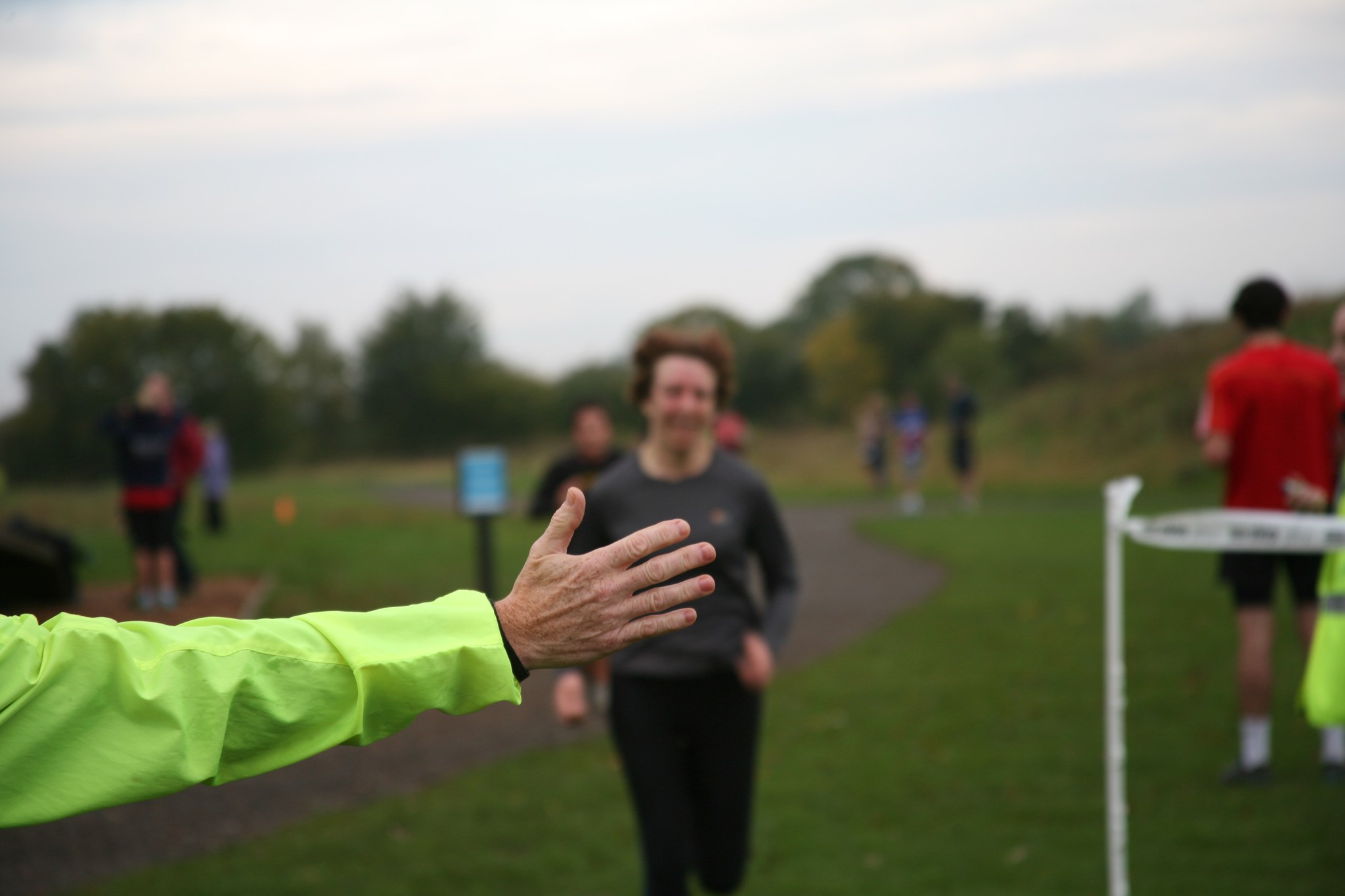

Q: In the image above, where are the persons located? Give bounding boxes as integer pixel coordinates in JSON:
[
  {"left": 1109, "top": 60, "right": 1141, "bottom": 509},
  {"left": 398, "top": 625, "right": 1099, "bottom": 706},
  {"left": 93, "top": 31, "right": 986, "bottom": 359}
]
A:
[
  {"left": 0, "top": 485, "right": 714, "bottom": 847},
  {"left": 856, "top": 386, "right": 893, "bottom": 503},
  {"left": 1201, "top": 278, "right": 1345, "bottom": 788},
  {"left": 197, "top": 416, "right": 230, "bottom": 537},
  {"left": 527, "top": 398, "right": 625, "bottom": 725},
  {"left": 896, "top": 387, "right": 928, "bottom": 519},
  {"left": 549, "top": 329, "right": 799, "bottom": 896},
  {"left": 943, "top": 371, "right": 982, "bottom": 507},
  {"left": 105, "top": 366, "right": 208, "bottom": 610}
]
